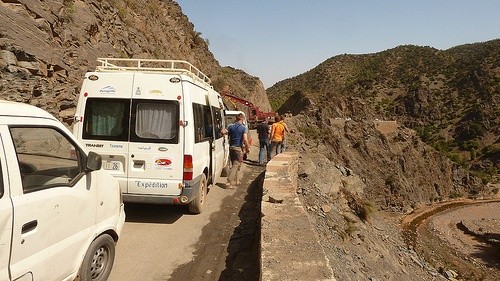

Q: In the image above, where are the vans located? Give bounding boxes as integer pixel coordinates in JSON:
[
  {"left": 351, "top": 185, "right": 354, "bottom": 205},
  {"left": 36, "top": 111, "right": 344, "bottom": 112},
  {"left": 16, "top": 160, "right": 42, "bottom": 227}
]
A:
[{"left": 73, "top": 57, "right": 230, "bottom": 213}]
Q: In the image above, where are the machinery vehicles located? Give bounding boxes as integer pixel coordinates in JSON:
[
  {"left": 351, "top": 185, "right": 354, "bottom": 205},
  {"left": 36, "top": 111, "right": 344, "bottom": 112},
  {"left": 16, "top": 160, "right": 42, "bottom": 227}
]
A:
[{"left": 218, "top": 89, "right": 279, "bottom": 129}]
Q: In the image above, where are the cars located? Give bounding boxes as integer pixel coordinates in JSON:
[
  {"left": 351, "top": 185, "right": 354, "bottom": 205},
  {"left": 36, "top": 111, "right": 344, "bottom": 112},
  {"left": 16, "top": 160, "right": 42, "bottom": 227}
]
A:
[
  {"left": 223, "top": 108, "right": 249, "bottom": 136},
  {"left": 0, "top": 97, "right": 126, "bottom": 281}
]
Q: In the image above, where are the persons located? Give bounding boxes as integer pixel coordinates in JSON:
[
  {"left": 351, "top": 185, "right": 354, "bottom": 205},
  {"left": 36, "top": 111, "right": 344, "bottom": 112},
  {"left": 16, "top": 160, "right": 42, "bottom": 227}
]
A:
[
  {"left": 221, "top": 114, "right": 251, "bottom": 189},
  {"left": 206, "top": 114, "right": 291, "bottom": 166}
]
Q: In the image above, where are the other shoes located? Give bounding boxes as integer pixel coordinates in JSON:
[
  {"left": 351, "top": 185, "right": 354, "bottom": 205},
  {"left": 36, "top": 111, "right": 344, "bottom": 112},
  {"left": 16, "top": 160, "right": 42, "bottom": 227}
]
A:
[{"left": 224, "top": 184, "right": 236, "bottom": 189}]
